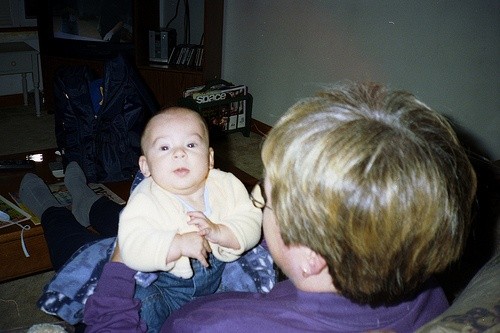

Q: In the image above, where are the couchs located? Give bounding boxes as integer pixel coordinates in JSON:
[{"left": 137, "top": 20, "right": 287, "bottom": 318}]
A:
[{"left": 411, "top": 252, "right": 500, "bottom": 333}]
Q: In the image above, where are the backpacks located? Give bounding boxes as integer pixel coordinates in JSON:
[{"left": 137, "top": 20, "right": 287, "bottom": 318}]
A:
[{"left": 48, "top": 59, "right": 158, "bottom": 182}]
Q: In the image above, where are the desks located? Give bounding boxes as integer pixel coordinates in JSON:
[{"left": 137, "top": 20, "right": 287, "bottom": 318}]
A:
[
  {"left": 0, "top": 43, "right": 41, "bottom": 118},
  {"left": 0, "top": 147, "right": 132, "bottom": 282}
]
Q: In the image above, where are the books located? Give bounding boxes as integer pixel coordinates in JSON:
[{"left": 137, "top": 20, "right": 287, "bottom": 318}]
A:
[{"left": 182, "top": 82, "right": 246, "bottom": 130}]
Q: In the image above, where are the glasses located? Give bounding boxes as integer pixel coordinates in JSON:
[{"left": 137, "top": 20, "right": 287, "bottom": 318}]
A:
[{"left": 249, "top": 179, "right": 275, "bottom": 212}]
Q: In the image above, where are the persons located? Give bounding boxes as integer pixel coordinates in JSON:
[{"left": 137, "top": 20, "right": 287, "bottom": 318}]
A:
[
  {"left": 118, "top": 108, "right": 263, "bottom": 333},
  {"left": 18, "top": 80, "right": 477, "bottom": 333}
]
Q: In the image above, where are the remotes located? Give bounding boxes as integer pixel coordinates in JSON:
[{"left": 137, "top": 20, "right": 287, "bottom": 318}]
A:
[{"left": 0, "top": 159, "right": 36, "bottom": 169}]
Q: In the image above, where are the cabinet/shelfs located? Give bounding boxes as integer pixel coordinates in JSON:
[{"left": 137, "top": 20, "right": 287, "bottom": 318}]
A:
[{"left": 132, "top": 1, "right": 224, "bottom": 108}]
